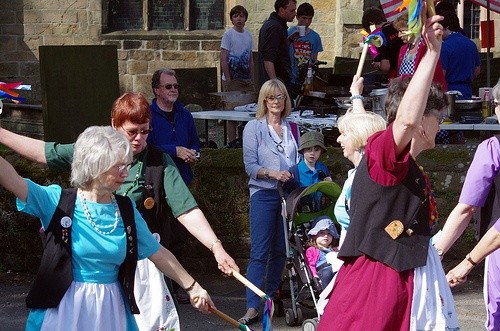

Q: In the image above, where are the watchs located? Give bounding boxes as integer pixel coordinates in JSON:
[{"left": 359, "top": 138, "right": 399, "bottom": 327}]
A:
[{"left": 434, "top": 244, "right": 444, "bottom": 256}]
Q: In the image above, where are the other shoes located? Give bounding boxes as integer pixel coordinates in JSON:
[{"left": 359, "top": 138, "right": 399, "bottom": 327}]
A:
[
  {"left": 175, "top": 286, "right": 191, "bottom": 304},
  {"left": 238, "top": 312, "right": 260, "bottom": 326}
]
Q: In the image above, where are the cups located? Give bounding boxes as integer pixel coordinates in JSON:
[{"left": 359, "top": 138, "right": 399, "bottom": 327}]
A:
[
  {"left": 443, "top": 91, "right": 458, "bottom": 116},
  {"left": 297, "top": 26, "right": 306, "bottom": 36},
  {"left": 370, "top": 89, "right": 389, "bottom": 120}
]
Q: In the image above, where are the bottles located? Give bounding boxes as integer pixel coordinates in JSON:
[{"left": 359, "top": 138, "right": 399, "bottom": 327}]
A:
[
  {"left": 482, "top": 89, "right": 494, "bottom": 121},
  {"left": 303, "top": 68, "right": 313, "bottom": 96}
]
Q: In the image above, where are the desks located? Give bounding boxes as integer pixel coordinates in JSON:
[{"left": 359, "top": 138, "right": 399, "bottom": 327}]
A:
[{"left": 188, "top": 108, "right": 500, "bottom": 147}]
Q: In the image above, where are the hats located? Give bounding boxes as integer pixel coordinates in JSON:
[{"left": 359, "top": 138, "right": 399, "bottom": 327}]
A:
[
  {"left": 297, "top": 132, "right": 327, "bottom": 156},
  {"left": 307, "top": 215, "right": 340, "bottom": 240}
]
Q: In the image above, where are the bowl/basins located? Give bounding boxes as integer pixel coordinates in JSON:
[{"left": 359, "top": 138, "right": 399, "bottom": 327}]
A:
[
  {"left": 454, "top": 100, "right": 483, "bottom": 110},
  {"left": 332, "top": 97, "right": 369, "bottom": 109},
  {"left": 309, "top": 92, "right": 326, "bottom": 98}
]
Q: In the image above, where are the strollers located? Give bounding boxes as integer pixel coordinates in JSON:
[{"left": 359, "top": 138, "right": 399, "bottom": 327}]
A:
[{"left": 277, "top": 170, "right": 342, "bottom": 331}]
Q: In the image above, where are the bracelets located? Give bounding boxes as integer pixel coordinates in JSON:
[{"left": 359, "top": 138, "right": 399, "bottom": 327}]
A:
[
  {"left": 265, "top": 169, "right": 269, "bottom": 179},
  {"left": 466, "top": 254, "right": 477, "bottom": 266},
  {"left": 185, "top": 280, "right": 196, "bottom": 292},
  {"left": 289, "top": 37, "right": 294, "bottom": 43},
  {"left": 351, "top": 95, "right": 364, "bottom": 103},
  {"left": 210, "top": 240, "right": 221, "bottom": 251}
]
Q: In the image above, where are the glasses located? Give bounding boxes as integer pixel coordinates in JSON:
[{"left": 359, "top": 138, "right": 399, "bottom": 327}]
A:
[
  {"left": 121, "top": 124, "right": 153, "bottom": 137},
  {"left": 161, "top": 83, "right": 182, "bottom": 89},
  {"left": 115, "top": 162, "right": 134, "bottom": 173},
  {"left": 265, "top": 94, "right": 287, "bottom": 103},
  {"left": 430, "top": 111, "right": 449, "bottom": 124},
  {"left": 491, "top": 99, "right": 500, "bottom": 110}
]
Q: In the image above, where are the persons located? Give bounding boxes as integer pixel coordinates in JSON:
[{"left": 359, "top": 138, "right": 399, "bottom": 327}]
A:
[
  {"left": 0, "top": 125, "right": 217, "bottom": 331},
  {"left": 316, "top": 3, "right": 450, "bottom": 331},
  {"left": 393, "top": 19, "right": 448, "bottom": 93},
  {"left": 283, "top": 131, "right": 332, "bottom": 213},
  {"left": 220, "top": 5, "right": 255, "bottom": 141},
  {"left": 148, "top": 69, "right": 202, "bottom": 303},
  {"left": 306, "top": 216, "right": 339, "bottom": 290},
  {"left": 433, "top": 77, "right": 500, "bottom": 331},
  {"left": 438, "top": 12, "right": 482, "bottom": 101},
  {"left": 334, "top": 76, "right": 460, "bottom": 330},
  {"left": 0, "top": 92, "right": 240, "bottom": 275},
  {"left": 287, "top": 2, "right": 323, "bottom": 84},
  {"left": 445, "top": 217, "right": 500, "bottom": 287},
  {"left": 362, "top": 7, "right": 406, "bottom": 87},
  {"left": 237, "top": 80, "right": 300, "bottom": 324},
  {"left": 259, "top": 0, "right": 300, "bottom": 92}
]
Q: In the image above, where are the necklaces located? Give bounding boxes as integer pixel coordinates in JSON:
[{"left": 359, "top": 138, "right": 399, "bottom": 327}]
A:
[
  {"left": 266, "top": 115, "right": 284, "bottom": 153},
  {"left": 76, "top": 189, "right": 119, "bottom": 235},
  {"left": 123, "top": 162, "right": 142, "bottom": 196}
]
